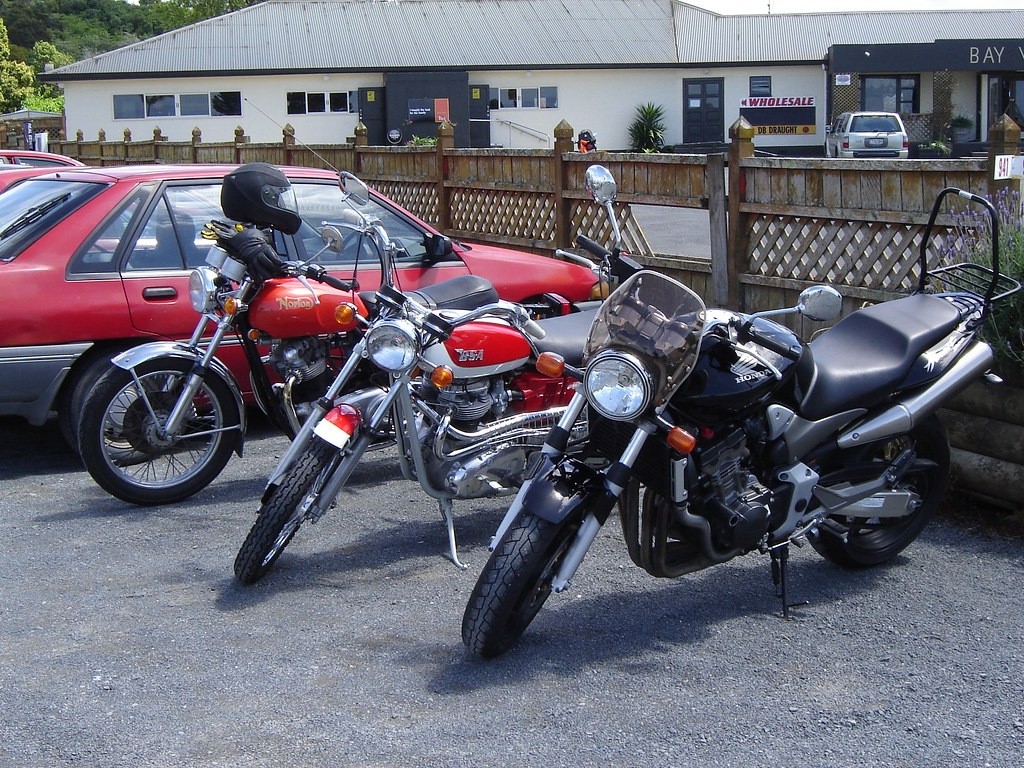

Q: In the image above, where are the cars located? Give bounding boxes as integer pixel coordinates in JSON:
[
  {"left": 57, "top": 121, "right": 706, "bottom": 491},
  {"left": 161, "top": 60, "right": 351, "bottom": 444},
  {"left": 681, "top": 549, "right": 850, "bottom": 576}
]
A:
[
  {"left": 0, "top": 157, "right": 611, "bottom": 472},
  {"left": 0, "top": 148, "right": 238, "bottom": 256},
  {"left": 823, "top": 110, "right": 915, "bottom": 161}
]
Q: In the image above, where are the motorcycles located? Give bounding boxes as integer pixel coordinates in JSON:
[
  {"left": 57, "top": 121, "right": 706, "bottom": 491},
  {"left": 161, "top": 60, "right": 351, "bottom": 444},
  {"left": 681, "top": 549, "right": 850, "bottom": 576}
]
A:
[
  {"left": 232, "top": 169, "right": 671, "bottom": 590},
  {"left": 459, "top": 172, "right": 1022, "bottom": 658},
  {"left": 75, "top": 160, "right": 500, "bottom": 507}
]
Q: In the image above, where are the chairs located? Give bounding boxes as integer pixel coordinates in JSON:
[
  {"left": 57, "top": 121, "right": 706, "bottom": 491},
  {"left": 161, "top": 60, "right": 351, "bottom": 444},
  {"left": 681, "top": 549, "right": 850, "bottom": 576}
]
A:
[{"left": 149, "top": 211, "right": 204, "bottom": 266}]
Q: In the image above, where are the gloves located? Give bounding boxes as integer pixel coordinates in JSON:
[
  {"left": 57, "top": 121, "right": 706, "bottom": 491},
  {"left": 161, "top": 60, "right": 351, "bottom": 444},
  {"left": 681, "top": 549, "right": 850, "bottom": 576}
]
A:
[
  {"left": 201, "top": 220, "right": 244, "bottom": 240},
  {"left": 227, "top": 229, "right": 283, "bottom": 282}
]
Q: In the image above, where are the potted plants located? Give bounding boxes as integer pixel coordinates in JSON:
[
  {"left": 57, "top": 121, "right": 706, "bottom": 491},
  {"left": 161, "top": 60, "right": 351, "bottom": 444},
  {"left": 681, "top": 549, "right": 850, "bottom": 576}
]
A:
[{"left": 916, "top": 115, "right": 976, "bottom": 158}]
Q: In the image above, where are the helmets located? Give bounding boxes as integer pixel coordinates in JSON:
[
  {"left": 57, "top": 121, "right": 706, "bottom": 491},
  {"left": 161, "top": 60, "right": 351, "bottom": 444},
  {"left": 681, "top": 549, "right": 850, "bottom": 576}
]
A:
[{"left": 220, "top": 162, "right": 303, "bottom": 235}]
